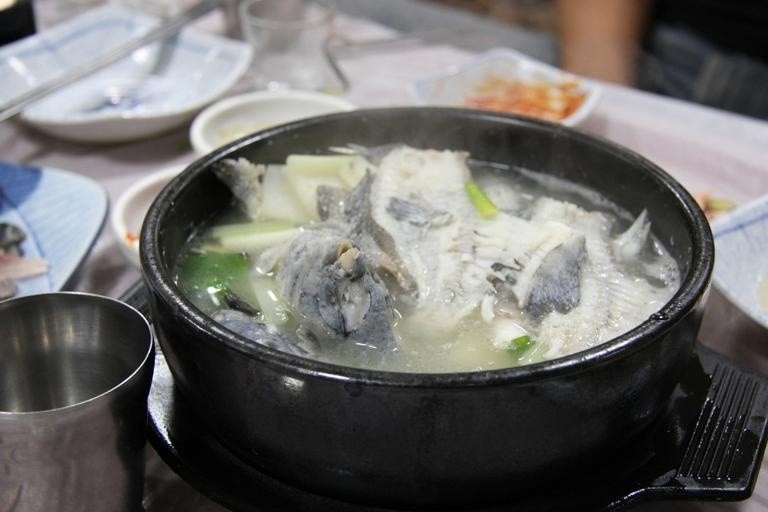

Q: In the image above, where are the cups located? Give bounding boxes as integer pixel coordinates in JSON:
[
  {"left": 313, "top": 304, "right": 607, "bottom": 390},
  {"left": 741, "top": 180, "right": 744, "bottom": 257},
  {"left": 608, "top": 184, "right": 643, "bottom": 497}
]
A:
[{"left": 0, "top": 293, "right": 156, "bottom": 512}]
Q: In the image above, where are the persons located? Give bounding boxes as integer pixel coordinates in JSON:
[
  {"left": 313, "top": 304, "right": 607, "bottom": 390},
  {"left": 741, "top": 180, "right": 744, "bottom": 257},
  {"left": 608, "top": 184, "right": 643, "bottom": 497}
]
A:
[{"left": 560, "top": 0, "right": 768, "bottom": 119}]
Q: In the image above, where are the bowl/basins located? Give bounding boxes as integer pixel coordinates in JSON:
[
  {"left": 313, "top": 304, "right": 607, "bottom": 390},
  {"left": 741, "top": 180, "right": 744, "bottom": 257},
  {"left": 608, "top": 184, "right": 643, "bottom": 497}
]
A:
[{"left": 140, "top": 104, "right": 718, "bottom": 504}]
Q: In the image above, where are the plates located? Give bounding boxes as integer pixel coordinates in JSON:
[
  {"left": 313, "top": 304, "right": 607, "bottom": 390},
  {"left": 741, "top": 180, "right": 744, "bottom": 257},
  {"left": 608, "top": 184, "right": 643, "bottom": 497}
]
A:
[
  {"left": 188, "top": 94, "right": 363, "bottom": 155},
  {"left": 416, "top": 49, "right": 606, "bottom": 128},
  {"left": 2, "top": 5, "right": 254, "bottom": 152},
  {"left": 100, "top": 277, "right": 768, "bottom": 512},
  {"left": 705, "top": 193, "right": 766, "bottom": 328},
  {"left": 1, "top": 163, "right": 108, "bottom": 298},
  {"left": 109, "top": 164, "right": 181, "bottom": 264}
]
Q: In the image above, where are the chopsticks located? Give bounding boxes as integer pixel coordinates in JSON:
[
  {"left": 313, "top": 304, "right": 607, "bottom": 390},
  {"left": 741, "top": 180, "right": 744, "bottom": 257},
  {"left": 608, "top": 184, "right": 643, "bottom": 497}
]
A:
[{"left": 1, "top": 5, "right": 243, "bottom": 128}]
